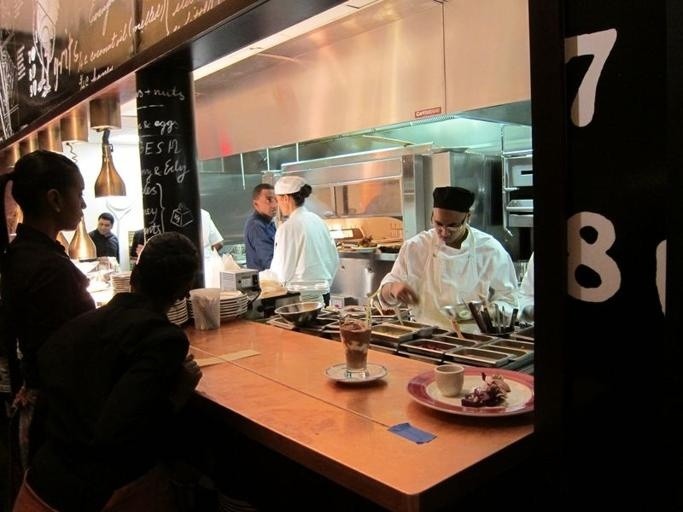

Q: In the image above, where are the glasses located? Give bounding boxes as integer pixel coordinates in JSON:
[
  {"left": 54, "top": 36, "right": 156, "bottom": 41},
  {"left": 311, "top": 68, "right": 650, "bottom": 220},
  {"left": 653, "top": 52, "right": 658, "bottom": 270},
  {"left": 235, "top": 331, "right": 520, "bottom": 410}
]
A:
[{"left": 429, "top": 210, "right": 468, "bottom": 231}]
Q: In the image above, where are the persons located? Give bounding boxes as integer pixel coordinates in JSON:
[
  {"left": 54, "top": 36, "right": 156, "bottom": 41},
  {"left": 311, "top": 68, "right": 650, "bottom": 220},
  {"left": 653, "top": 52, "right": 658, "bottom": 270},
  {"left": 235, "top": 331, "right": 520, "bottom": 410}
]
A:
[
  {"left": 88, "top": 212, "right": 120, "bottom": 265},
  {"left": 519, "top": 250, "right": 535, "bottom": 321},
  {"left": 131, "top": 228, "right": 145, "bottom": 257},
  {"left": 1, "top": 148, "right": 95, "bottom": 473},
  {"left": 243, "top": 183, "right": 280, "bottom": 272},
  {"left": 200, "top": 208, "right": 226, "bottom": 289},
  {"left": 376, "top": 186, "right": 519, "bottom": 329},
  {"left": 258, "top": 175, "right": 340, "bottom": 308},
  {"left": 7, "top": 231, "right": 203, "bottom": 512}
]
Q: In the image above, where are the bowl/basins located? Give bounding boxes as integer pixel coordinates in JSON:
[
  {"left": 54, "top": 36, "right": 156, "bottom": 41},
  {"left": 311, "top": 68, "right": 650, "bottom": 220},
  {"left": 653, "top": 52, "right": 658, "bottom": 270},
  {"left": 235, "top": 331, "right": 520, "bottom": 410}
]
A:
[{"left": 273, "top": 301, "right": 326, "bottom": 325}]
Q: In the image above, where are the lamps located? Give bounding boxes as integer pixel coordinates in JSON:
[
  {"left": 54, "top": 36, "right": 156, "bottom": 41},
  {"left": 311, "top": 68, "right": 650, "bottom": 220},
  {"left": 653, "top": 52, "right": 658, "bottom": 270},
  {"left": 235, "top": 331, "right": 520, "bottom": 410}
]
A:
[
  {"left": 68, "top": 214, "right": 97, "bottom": 261},
  {"left": 56, "top": 232, "right": 69, "bottom": 256},
  {"left": 94, "top": 128, "right": 127, "bottom": 197}
]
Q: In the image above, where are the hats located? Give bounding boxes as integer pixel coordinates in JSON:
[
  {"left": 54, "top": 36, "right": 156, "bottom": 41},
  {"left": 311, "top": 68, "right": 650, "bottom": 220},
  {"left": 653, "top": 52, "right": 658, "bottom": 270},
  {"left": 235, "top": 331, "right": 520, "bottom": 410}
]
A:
[
  {"left": 272, "top": 174, "right": 307, "bottom": 196},
  {"left": 432, "top": 185, "right": 475, "bottom": 213}
]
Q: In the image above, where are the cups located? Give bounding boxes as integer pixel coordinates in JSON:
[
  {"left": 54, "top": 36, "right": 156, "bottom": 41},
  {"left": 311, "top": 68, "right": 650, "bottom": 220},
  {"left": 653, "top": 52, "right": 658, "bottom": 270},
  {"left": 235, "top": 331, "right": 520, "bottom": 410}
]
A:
[
  {"left": 433, "top": 363, "right": 466, "bottom": 398},
  {"left": 188, "top": 286, "right": 221, "bottom": 331},
  {"left": 337, "top": 304, "right": 373, "bottom": 378}
]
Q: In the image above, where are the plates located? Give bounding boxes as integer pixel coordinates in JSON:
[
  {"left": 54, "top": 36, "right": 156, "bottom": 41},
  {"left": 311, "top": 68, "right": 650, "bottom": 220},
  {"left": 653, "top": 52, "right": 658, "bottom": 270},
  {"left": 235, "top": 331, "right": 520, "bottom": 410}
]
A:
[
  {"left": 351, "top": 244, "right": 381, "bottom": 253},
  {"left": 109, "top": 270, "right": 248, "bottom": 326},
  {"left": 403, "top": 365, "right": 537, "bottom": 423},
  {"left": 324, "top": 363, "right": 387, "bottom": 385}
]
